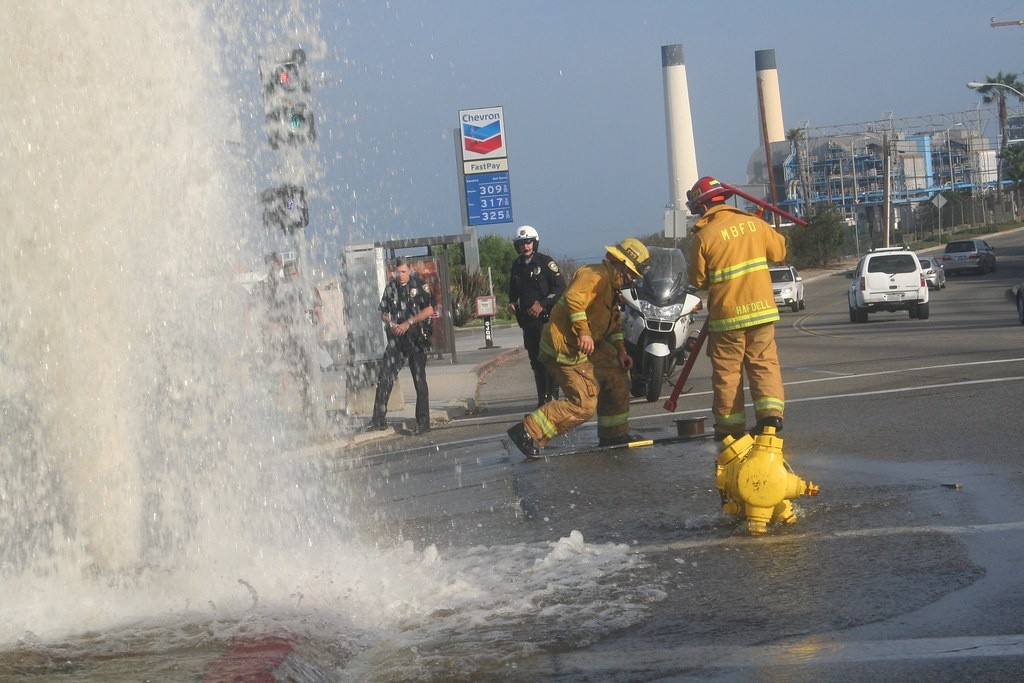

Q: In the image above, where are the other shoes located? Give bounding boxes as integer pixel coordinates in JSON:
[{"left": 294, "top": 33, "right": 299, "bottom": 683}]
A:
[
  {"left": 749, "top": 416, "right": 783, "bottom": 436},
  {"left": 363, "top": 418, "right": 388, "bottom": 431},
  {"left": 714, "top": 432, "right": 745, "bottom": 440},
  {"left": 538, "top": 402, "right": 545, "bottom": 407},
  {"left": 412, "top": 423, "right": 430, "bottom": 436}
]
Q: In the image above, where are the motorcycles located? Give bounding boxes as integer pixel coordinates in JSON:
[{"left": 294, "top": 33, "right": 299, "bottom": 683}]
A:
[{"left": 617, "top": 245, "right": 703, "bottom": 402}]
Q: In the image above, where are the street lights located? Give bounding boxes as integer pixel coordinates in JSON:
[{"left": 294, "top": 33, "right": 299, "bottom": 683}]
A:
[{"left": 946, "top": 123, "right": 963, "bottom": 192}]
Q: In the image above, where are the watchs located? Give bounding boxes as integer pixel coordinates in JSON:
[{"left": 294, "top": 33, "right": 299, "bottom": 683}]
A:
[{"left": 406, "top": 318, "right": 414, "bottom": 326}]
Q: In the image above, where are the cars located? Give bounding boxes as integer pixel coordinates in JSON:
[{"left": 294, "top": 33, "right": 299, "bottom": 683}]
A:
[{"left": 915, "top": 255, "right": 946, "bottom": 291}]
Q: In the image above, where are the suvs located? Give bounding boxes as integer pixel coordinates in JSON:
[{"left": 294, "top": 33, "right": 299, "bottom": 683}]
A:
[
  {"left": 768, "top": 263, "right": 806, "bottom": 312},
  {"left": 846, "top": 246, "right": 930, "bottom": 323},
  {"left": 943, "top": 238, "right": 997, "bottom": 278}
]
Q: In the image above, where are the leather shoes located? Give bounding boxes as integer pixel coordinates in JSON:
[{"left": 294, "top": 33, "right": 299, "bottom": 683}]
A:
[
  {"left": 507, "top": 423, "right": 539, "bottom": 455},
  {"left": 598, "top": 434, "right": 644, "bottom": 447}
]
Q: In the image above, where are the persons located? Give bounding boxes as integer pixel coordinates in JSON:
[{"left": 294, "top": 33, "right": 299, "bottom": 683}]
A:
[
  {"left": 685, "top": 176, "right": 787, "bottom": 441},
  {"left": 507, "top": 225, "right": 568, "bottom": 418},
  {"left": 356, "top": 255, "right": 436, "bottom": 436},
  {"left": 250, "top": 251, "right": 338, "bottom": 394},
  {"left": 507, "top": 237, "right": 654, "bottom": 458}
]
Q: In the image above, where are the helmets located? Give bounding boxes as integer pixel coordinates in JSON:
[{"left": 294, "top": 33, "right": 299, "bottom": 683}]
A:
[
  {"left": 513, "top": 226, "right": 539, "bottom": 254},
  {"left": 605, "top": 238, "right": 651, "bottom": 278},
  {"left": 685, "top": 176, "right": 734, "bottom": 215}
]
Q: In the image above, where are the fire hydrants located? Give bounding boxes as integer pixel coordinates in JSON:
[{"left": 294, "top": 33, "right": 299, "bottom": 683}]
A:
[{"left": 714, "top": 425, "right": 820, "bottom": 537}]
